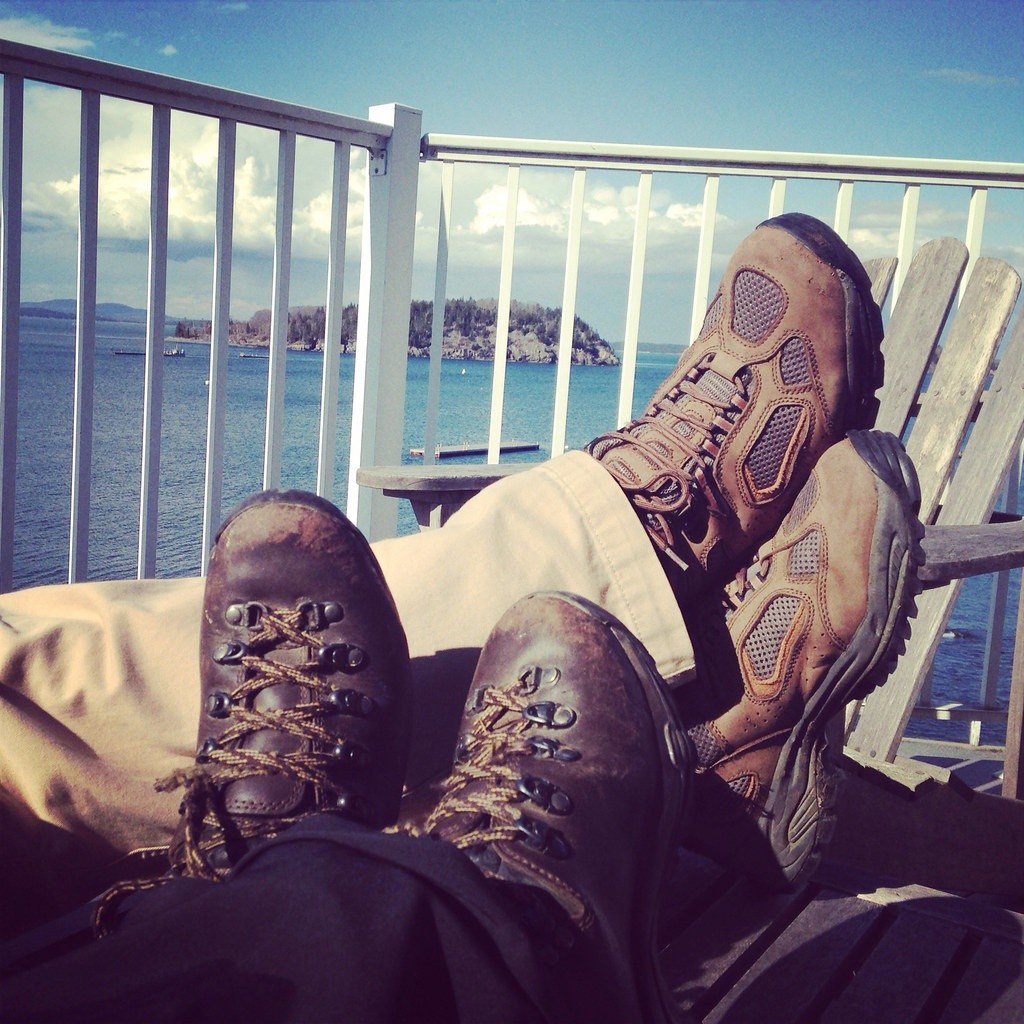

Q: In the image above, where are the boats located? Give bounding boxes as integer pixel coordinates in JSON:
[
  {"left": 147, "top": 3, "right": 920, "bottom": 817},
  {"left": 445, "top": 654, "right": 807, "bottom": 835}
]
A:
[
  {"left": 941, "top": 629, "right": 970, "bottom": 638},
  {"left": 237, "top": 353, "right": 270, "bottom": 359},
  {"left": 113, "top": 346, "right": 187, "bottom": 358}
]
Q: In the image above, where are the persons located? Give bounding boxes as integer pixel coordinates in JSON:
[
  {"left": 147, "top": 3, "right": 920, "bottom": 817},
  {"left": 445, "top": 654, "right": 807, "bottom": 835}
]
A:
[
  {"left": 1, "top": 490, "right": 702, "bottom": 1024},
  {"left": 1, "top": 212, "right": 926, "bottom": 896}
]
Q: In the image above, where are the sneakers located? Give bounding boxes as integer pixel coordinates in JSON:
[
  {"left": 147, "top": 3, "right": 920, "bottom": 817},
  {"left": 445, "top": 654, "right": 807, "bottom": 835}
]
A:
[
  {"left": 583, "top": 212, "right": 885, "bottom": 619},
  {"left": 664, "top": 428, "right": 926, "bottom": 897}
]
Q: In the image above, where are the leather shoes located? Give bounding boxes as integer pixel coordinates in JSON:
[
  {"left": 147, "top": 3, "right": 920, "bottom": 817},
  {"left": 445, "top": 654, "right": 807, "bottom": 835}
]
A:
[
  {"left": 90, "top": 496, "right": 414, "bottom": 941},
  {"left": 382, "top": 589, "right": 693, "bottom": 1024}
]
